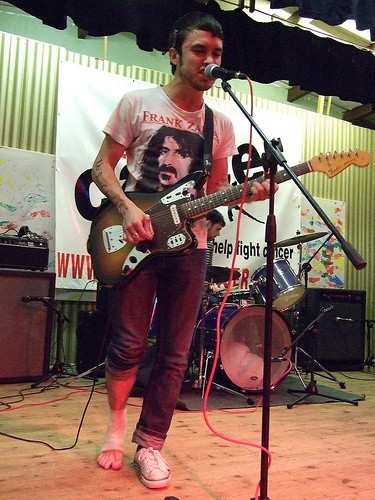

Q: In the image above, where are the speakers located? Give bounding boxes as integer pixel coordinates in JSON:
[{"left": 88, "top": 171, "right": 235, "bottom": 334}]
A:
[
  {"left": 75, "top": 309, "right": 105, "bottom": 380},
  {"left": 0, "top": 267, "right": 56, "bottom": 383},
  {"left": 285, "top": 287, "right": 366, "bottom": 373}
]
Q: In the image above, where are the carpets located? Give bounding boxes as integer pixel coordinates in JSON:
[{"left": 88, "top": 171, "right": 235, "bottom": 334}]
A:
[{"left": 131, "top": 374, "right": 365, "bottom": 411}]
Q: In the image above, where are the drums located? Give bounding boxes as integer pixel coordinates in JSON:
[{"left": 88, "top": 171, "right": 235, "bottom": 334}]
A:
[
  {"left": 250, "top": 257, "right": 306, "bottom": 313},
  {"left": 192, "top": 301, "right": 294, "bottom": 392},
  {"left": 217, "top": 287, "right": 254, "bottom": 302}
]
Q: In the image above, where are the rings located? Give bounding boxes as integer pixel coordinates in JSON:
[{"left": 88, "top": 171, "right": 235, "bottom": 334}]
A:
[{"left": 133, "top": 233, "right": 138, "bottom": 239}]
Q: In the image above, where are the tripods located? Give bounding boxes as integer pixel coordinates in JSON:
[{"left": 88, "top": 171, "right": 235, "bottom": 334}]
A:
[
  {"left": 31, "top": 301, "right": 99, "bottom": 389},
  {"left": 182, "top": 290, "right": 256, "bottom": 406},
  {"left": 279, "top": 243, "right": 359, "bottom": 410}
]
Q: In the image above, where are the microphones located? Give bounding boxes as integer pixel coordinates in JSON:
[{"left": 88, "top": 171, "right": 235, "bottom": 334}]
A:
[
  {"left": 270, "top": 357, "right": 287, "bottom": 362},
  {"left": 21, "top": 295, "right": 53, "bottom": 303},
  {"left": 204, "top": 64, "right": 247, "bottom": 79},
  {"left": 335, "top": 316, "right": 355, "bottom": 322}
]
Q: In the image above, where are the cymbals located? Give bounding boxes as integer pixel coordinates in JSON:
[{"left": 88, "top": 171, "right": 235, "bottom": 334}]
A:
[
  {"left": 274, "top": 229, "right": 328, "bottom": 249},
  {"left": 204, "top": 262, "right": 243, "bottom": 284}
]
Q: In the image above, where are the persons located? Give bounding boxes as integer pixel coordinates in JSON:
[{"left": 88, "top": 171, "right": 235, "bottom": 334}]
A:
[
  {"left": 91, "top": 11, "right": 278, "bottom": 489},
  {"left": 206, "top": 210, "right": 225, "bottom": 280}
]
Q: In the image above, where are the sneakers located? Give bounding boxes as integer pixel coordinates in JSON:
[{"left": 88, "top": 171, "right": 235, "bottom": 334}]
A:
[{"left": 133, "top": 447, "right": 172, "bottom": 488}]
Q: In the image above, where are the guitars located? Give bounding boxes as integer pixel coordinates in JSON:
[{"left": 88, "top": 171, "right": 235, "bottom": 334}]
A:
[{"left": 88, "top": 146, "right": 372, "bottom": 288}]
